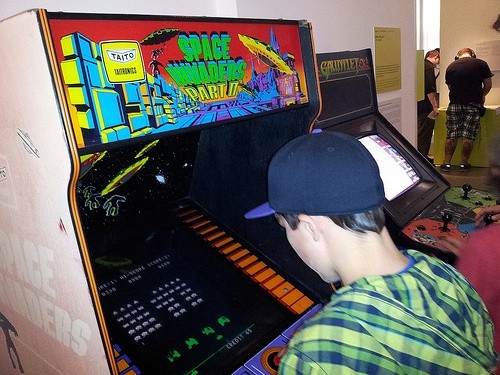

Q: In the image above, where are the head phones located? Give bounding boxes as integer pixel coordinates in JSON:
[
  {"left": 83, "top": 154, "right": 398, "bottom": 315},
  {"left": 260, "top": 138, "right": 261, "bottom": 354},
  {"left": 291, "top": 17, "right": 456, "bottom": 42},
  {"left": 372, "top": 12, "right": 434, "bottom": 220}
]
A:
[{"left": 455, "top": 51, "right": 476, "bottom": 60}]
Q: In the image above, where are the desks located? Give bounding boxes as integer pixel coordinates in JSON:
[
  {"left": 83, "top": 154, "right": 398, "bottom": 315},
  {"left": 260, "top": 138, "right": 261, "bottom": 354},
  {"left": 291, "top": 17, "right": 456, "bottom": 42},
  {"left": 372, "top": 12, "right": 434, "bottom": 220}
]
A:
[{"left": 434, "top": 106, "right": 500, "bottom": 167}]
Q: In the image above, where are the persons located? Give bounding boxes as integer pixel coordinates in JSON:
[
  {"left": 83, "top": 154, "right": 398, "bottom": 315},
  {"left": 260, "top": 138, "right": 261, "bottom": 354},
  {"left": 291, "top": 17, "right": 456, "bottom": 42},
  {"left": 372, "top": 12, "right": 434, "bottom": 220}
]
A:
[
  {"left": 417, "top": 50, "right": 439, "bottom": 164},
  {"left": 245, "top": 129, "right": 500, "bottom": 375},
  {"left": 440, "top": 48, "right": 495, "bottom": 173},
  {"left": 433, "top": 48, "right": 440, "bottom": 106},
  {"left": 436, "top": 136, "right": 500, "bottom": 375}
]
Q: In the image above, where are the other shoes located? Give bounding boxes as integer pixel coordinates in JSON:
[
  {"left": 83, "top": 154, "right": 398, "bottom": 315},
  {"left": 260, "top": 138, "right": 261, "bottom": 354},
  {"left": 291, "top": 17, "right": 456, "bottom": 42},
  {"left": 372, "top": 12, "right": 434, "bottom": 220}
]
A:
[
  {"left": 459, "top": 163, "right": 471, "bottom": 171},
  {"left": 440, "top": 163, "right": 451, "bottom": 172}
]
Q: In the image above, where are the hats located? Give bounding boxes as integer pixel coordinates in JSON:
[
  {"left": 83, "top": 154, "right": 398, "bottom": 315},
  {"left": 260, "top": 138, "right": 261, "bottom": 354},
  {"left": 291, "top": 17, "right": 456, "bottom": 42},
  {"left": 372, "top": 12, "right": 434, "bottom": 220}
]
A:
[{"left": 244, "top": 128, "right": 386, "bottom": 219}]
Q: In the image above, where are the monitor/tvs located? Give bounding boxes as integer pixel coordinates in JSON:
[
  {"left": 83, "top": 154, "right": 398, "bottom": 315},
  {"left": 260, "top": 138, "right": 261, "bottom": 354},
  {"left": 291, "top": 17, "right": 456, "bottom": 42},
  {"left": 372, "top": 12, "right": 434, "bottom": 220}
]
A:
[
  {"left": 357, "top": 134, "right": 420, "bottom": 201},
  {"left": 83, "top": 196, "right": 318, "bottom": 375}
]
